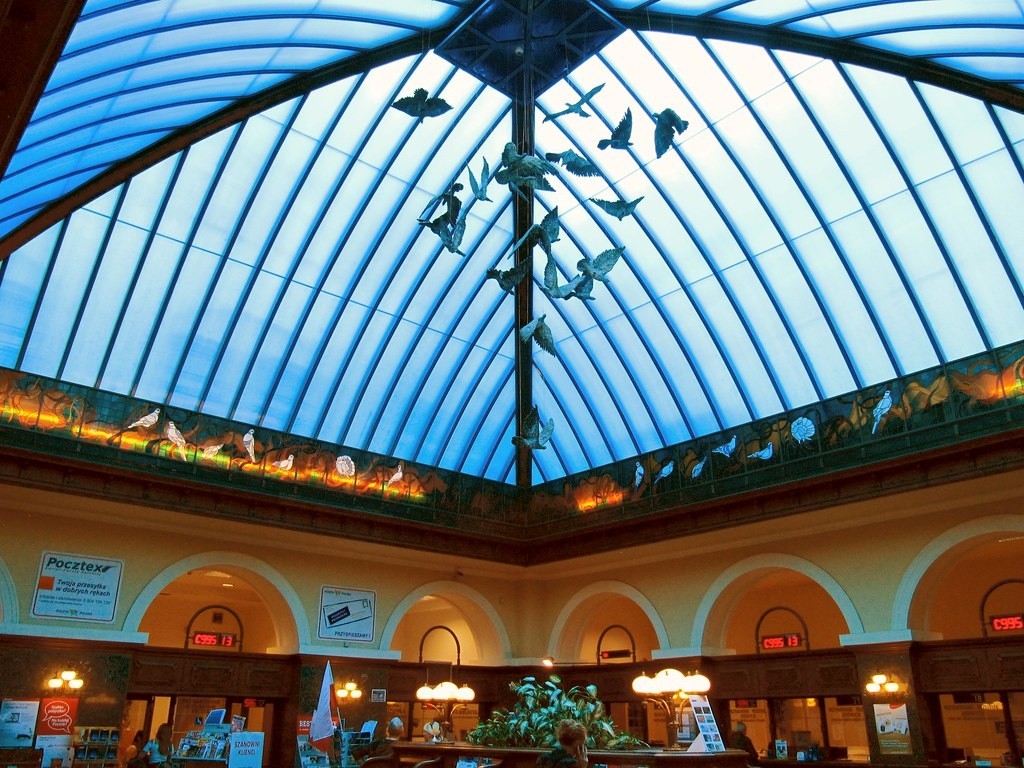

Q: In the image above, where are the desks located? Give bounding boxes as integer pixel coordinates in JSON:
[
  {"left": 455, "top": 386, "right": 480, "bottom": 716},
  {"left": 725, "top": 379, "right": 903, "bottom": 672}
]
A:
[{"left": 170, "top": 756, "right": 229, "bottom": 768}]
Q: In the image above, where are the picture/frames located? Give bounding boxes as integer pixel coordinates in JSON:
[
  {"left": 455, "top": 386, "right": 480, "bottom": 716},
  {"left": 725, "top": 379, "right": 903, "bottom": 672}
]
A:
[{"left": 371, "top": 688, "right": 387, "bottom": 702}]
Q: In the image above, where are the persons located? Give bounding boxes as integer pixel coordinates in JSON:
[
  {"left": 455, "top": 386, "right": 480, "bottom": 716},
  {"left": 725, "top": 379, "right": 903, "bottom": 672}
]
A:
[
  {"left": 534, "top": 719, "right": 587, "bottom": 768},
  {"left": 726, "top": 722, "right": 758, "bottom": 759},
  {"left": 351, "top": 717, "right": 403, "bottom": 766},
  {"left": 423, "top": 722, "right": 443, "bottom": 742},
  {"left": 122, "top": 723, "right": 174, "bottom": 768}
]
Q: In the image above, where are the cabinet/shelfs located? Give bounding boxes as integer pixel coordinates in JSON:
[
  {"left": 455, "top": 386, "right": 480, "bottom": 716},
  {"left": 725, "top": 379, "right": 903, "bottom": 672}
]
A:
[{"left": 73, "top": 727, "right": 118, "bottom": 768}]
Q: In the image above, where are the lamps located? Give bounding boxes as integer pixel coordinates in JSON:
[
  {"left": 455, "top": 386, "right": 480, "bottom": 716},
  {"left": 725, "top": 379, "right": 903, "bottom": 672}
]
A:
[
  {"left": 866, "top": 671, "right": 898, "bottom": 693},
  {"left": 416, "top": 681, "right": 474, "bottom": 744},
  {"left": 543, "top": 657, "right": 555, "bottom": 666},
  {"left": 48, "top": 664, "right": 83, "bottom": 689},
  {"left": 632, "top": 668, "right": 711, "bottom": 751},
  {"left": 337, "top": 678, "right": 362, "bottom": 698}
]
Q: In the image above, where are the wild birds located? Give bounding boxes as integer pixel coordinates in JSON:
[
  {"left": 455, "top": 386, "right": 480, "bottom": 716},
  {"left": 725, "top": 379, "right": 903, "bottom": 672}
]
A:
[
  {"left": 589, "top": 195, "right": 644, "bottom": 221},
  {"left": 597, "top": 108, "right": 633, "bottom": 150},
  {"left": 652, "top": 108, "right": 689, "bottom": 159},
  {"left": 415, "top": 142, "right": 626, "bottom": 300},
  {"left": 519, "top": 315, "right": 557, "bottom": 359},
  {"left": 390, "top": 88, "right": 452, "bottom": 123},
  {"left": 542, "top": 83, "right": 605, "bottom": 123},
  {"left": 511, "top": 405, "right": 555, "bottom": 449}
]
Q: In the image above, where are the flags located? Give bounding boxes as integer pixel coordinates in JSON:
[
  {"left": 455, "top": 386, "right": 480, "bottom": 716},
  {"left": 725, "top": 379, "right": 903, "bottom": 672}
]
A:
[{"left": 309, "top": 660, "right": 339, "bottom": 761}]
